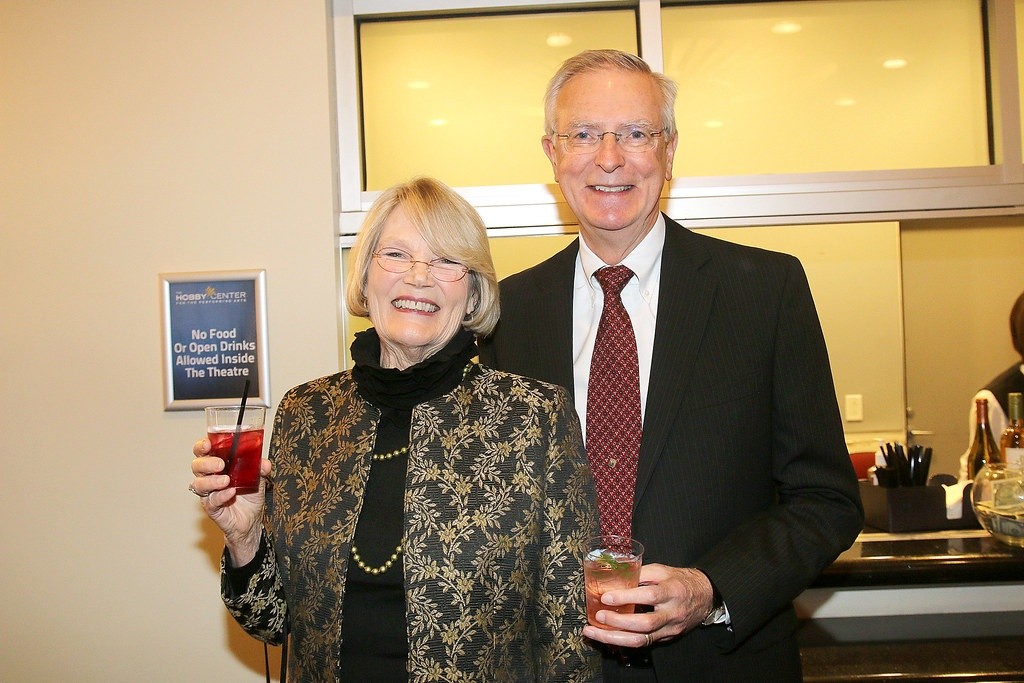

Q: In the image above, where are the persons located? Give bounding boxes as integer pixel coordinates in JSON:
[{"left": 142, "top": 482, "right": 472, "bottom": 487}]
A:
[
  {"left": 189, "top": 174, "right": 609, "bottom": 683},
  {"left": 957, "top": 294, "right": 1024, "bottom": 480},
  {"left": 477, "top": 48, "right": 865, "bottom": 683}
]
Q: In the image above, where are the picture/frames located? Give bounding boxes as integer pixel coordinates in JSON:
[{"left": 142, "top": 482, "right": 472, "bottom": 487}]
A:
[{"left": 159, "top": 269, "right": 271, "bottom": 412}]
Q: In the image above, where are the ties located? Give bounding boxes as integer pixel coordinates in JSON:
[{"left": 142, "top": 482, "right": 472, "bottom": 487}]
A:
[{"left": 586, "top": 265, "right": 643, "bottom": 550}]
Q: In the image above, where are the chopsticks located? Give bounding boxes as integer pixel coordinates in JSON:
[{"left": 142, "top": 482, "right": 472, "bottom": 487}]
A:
[{"left": 876, "top": 441, "right": 934, "bottom": 486}]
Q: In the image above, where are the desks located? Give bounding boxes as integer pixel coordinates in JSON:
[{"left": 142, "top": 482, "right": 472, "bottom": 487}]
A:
[{"left": 828, "top": 531, "right": 1024, "bottom": 587}]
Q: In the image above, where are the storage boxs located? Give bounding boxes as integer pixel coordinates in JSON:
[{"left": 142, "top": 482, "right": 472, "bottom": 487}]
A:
[{"left": 859, "top": 480, "right": 948, "bottom": 534}]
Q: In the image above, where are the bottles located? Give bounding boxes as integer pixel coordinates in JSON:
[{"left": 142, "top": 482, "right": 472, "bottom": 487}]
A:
[
  {"left": 1000, "top": 391, "right": 1024, "bottom": 465},
  {"left": 968, "top": 400, "right": 1001, "bottom": 480}
]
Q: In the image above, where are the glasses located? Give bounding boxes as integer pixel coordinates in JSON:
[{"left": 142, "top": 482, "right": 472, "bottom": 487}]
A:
[
  {"left": 373, "top": 247, "right": 474, "bottom": 282},
  {"left": 556, "top": 126, "right": 665, "bottom": 152}
]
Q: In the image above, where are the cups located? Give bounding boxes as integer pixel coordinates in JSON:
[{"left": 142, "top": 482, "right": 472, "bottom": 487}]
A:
[
  {"left": 583, "top": 535, "right": 645, "bottom": 631},
  {"left": 205, "top": 405, "right": 266, "bottom": 494}
]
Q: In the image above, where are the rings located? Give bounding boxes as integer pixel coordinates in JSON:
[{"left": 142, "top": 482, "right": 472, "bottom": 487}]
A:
[
  {"left": 189, "top": 488, "right": 209, "bottom": 497},
  {"left": 645, "top": 634, "right": 653, "bottom": 646}
]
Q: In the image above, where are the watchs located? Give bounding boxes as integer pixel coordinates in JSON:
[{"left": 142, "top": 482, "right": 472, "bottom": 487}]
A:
[{"left": 698, "top": 568, "right": 725, "bottom": 626}]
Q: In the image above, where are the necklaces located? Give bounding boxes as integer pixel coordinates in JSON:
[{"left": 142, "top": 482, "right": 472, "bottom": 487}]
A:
[{"left": 347, "top": 352, "right": 477, "bottom": 577}]
